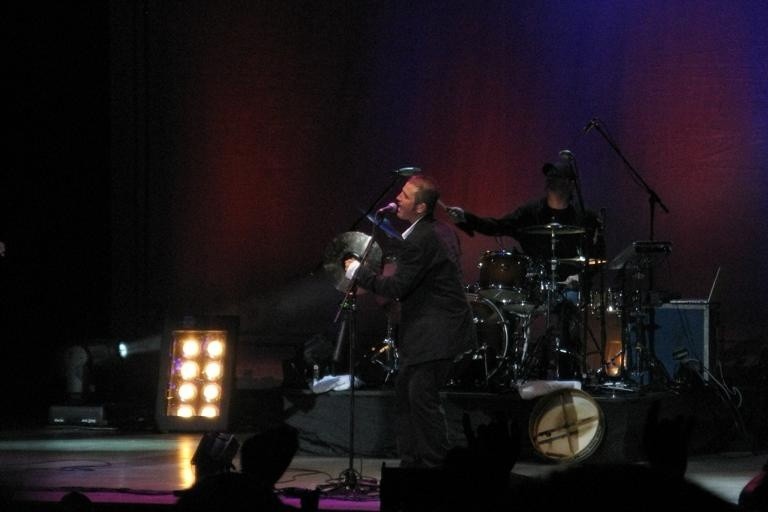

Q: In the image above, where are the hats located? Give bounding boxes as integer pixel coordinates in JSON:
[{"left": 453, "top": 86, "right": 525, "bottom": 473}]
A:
[{"left": 542, "top": 159, "right": 578, "bottom": 177}]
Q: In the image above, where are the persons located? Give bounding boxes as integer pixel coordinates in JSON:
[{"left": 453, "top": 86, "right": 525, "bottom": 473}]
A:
[
  {"left": 446, "top": 153, "right": 606, "bottom": 381},
  {"left": 343, "top": 173, "right": 476, "bottom": 472}
]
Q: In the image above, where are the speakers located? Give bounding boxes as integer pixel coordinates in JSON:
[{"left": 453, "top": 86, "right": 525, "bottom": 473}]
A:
[{"left": 624, "top": 305, "right": 716, "bottom": 384}]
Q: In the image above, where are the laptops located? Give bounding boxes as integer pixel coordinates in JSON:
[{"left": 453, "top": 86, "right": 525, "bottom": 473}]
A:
[{"left": 666, "top": 263, "right": 724, "bottom": 304}]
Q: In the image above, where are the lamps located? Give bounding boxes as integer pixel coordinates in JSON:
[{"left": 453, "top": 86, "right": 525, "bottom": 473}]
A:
[{"left": 156, "top": 314, "right": 240, "bottom": 433}]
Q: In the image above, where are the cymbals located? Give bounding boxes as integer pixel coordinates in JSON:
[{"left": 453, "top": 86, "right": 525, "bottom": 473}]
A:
[
  {"left": 358, "top": 207, "right": 402, "bottom": 241},
  {"left": 323, "top": 232, "right": 384, "bottom": 296},
  {"left": 557, "top": 257, "right": 606, "bottom": 265},
  {"left": 523, "top": 226, "right": 585, "bottom": 234}
]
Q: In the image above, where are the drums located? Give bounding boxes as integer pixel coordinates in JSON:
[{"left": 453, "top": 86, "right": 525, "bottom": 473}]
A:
[
  {"left": 579, "top": 287, "right": 622, "bottom": 314},
  {"left": 479, "top": 249, "right": 531, "bottom": 302},
  {"left": 502, "top": 272, "right": 549, "bottom": 319},
  {"left": 542, "top": 334, "right": 583, "bottom": 369},
  {"left": 447, "top": 291, "right": 528, "bottom": 388},
  {"left": 529, "top": 388, "right": 605, "bottom": 464}
]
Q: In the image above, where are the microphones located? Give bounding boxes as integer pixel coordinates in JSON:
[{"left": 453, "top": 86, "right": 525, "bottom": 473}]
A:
[
  {"left": 582, "top": 115, "right": 599, "bottom": 132},
  {"left": 395, "top": 165, "right": 423, "bottom": 178},
  {"left": 377, "top": 200, "right": 397, "bottom": 215}
]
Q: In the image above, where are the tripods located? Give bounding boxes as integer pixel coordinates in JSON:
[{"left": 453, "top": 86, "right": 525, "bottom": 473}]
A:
[
  {"left": 319, "top": 232, "right": 378, "bottom": 500},
  {"left": 516, "top": 241, "right": 645, "bottom": 381}
]
